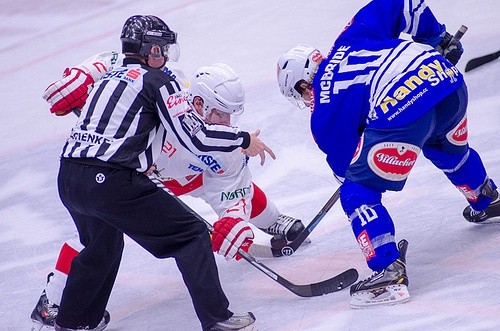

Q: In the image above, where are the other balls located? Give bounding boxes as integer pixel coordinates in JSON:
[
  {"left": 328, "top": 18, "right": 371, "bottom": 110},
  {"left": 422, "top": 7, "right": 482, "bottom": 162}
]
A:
[{"left": 270, "top": 235, "right": 286, "bottom": 248}]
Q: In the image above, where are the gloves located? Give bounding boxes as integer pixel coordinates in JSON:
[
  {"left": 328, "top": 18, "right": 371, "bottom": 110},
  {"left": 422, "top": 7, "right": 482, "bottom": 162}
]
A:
[
  {"left": 435, "top": 32, "right": 464, "bottom": 67},
  {"left": 210, "top": 218, "right": 255, "bottom": 262},
  {"left": 42, "top": 68, "right": 95, "bottom": 116}
]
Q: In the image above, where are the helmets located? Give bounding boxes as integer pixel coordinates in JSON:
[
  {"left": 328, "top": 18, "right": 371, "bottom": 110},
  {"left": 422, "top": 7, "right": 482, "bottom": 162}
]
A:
[
  {"left": 187, "top": 63, "right": 245, "bottom": 126},
  {"left": 276, "top": 44, "right": 323, "bottom": 110},
  {"left": 120, "top": 14, "right": 178, "bottom": 61}
]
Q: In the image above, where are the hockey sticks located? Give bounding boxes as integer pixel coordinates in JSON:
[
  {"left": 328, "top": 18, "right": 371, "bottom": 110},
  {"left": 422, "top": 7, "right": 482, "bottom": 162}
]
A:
[
  {"left": 74, "top": 108, "right": 359, "bottom": 298},
  {"left": 246, "top": 25, "right": 468, "bottom": 257}
]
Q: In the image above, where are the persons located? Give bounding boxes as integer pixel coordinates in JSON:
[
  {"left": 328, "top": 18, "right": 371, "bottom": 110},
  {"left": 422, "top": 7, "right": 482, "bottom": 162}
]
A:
[
  {"left": 55, "top": 15, "right": 256, "bottom": 331},
  {"left": 277, "top": 0, "right": 500, "bottom": 308},
  {"left": 30, "top": 49, "right": 311, "bottom": 331}
]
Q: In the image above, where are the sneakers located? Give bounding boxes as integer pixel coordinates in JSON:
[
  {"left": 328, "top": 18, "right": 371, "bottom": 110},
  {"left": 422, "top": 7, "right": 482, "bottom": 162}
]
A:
[
  {"left": 30, "top": 272, "right": 61, "bottom": 328},
  {"left": 258, "top": 213, "right": 313, "bottom": 246},
  {"left": 203, "top": 312, "right": 258, "bottom": 331},
  {"left": 53, "top": 309, "right": 111, "bottom": 331},
  {"left": 349, "top": 239, "right": 409, "bottom": 309},
  {"left": 463, "top": 175, "right": 500, "bottom": 225}
]
